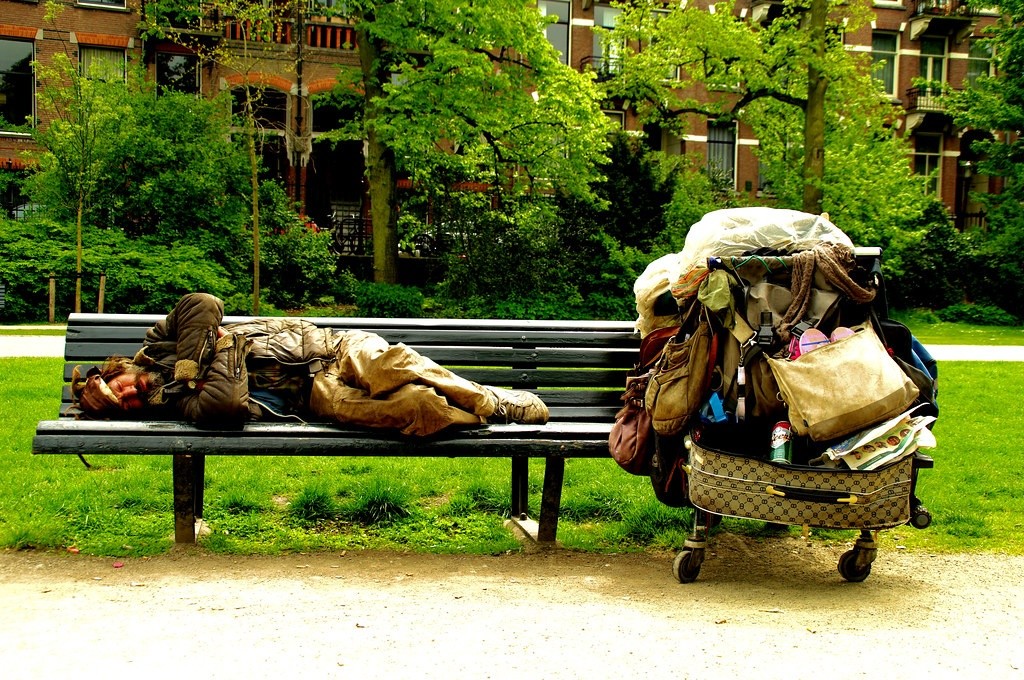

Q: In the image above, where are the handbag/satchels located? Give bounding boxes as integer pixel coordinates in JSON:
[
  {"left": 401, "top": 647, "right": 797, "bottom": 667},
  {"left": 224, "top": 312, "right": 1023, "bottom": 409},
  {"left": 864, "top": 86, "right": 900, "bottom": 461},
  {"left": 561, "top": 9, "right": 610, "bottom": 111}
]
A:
[
  {"left": 642, "top": 439, "right": 695, "bottom": 508},
  {"left": 768, "top": 320, "right": 920, "bottom": 442},
  {"left": 744, "top": 344, "right": 790, "bottom": 417},
  {"left": 644, "top": 322, "right": 711, "bottom": 437},
  {"left": 893, "top": 332, "right": 939, "bottom": 431},
  {"left": 606, "top": 370, "right": 657, "bottom": 475}
]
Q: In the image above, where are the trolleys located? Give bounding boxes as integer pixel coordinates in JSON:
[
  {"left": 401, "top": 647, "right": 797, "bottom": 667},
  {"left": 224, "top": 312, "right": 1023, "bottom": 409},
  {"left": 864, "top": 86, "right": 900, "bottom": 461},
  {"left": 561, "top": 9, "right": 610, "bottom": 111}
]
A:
[{"left": 672, "top": 243, "right": 931, "bottom": 584}]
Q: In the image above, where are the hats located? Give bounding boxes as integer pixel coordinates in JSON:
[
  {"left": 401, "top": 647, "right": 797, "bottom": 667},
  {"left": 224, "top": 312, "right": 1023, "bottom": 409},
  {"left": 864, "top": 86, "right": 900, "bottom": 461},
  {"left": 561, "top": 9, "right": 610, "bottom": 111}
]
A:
[{"left": 80, "top": 374, "right": 124, "bottom": 418}]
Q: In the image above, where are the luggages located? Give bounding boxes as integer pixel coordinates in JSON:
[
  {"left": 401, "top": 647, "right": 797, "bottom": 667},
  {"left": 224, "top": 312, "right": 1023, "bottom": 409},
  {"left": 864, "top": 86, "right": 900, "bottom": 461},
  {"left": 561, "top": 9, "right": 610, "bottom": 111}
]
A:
[{"left": 677, "top": 424, "right": 933, "bottom": 530}]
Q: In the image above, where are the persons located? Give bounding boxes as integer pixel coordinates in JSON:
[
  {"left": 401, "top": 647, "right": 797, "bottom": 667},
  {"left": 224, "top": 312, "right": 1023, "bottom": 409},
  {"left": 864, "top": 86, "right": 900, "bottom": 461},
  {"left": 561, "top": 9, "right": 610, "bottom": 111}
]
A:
[{"left": 67, "top": 293, "right": 549, "bottom": 437}]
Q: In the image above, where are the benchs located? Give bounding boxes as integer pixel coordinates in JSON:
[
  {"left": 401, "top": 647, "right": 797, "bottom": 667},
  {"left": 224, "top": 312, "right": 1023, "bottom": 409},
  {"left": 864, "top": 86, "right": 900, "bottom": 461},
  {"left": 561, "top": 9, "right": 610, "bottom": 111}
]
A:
[{"left": 33, "top": 312, "right": 642, "bottom": 546}]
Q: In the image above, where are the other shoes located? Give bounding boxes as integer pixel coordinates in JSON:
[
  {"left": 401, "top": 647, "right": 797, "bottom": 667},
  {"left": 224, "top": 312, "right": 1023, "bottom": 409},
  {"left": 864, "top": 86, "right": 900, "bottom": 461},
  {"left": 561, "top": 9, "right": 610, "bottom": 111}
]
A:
[{"left": 483, "top": 384, "right": 550, "bottom": 426}]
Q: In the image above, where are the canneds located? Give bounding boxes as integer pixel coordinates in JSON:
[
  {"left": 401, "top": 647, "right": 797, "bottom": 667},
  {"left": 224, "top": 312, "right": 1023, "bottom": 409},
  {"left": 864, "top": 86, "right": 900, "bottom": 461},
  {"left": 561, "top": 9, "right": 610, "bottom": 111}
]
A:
[{"left": 769, "top": 420, "right": 793, "bottom": 466}]
